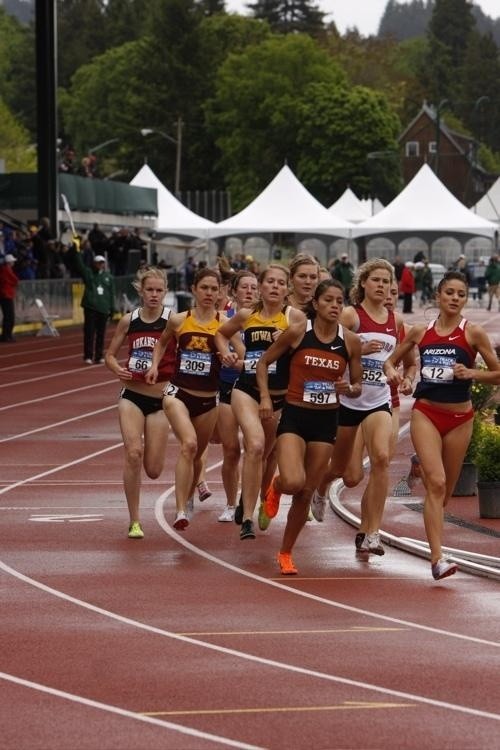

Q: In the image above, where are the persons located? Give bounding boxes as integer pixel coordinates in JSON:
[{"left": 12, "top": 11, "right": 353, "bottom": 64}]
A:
[
  {"left": 104, "top": 269, "right": 179, "bottom": 538},
  {"left": 214, "top": 266, "right": 307, "bottom": 538},
  {"left": 355, "top": 264, "right": 418, "bottom": 552},
  {"left": 446, "top": 257, "right": 467, "bottom": 277},
  {"left": 282, "top": 255, "right": 320, "bottom": 323},
  {"left": 412, "top": 250, "right": 437, "bottom": 301},
  {"left": 383, "top": 272, "right": 500, "bottom": 581},
  {"left": 78, "top": 222, "right": 151, "bottom": 265},
  {"left": 0, "top": 221, "right": 68, "bottom": 280},
  {"left": 399, "top": 261, "right": 415, "bottom": 314},
  {"left": 0, "top": 253, "right": 20, "bottom": 344},
  {"left": 319, "top": 253, "right": 359, "bottom": 289},
  {"left": 146, "top": 270, "right": 243, "bottom": 530},
  {"left": 256, "top": 285, "right": 362, "bottom": 576},
  {"left": 311, "top": 258, "right": 404, "bottom": 556},
  {"left": 220, "top": 273, "right": 260, "bottom": 526},
  {"left": 71, "top": 239, "right": 113, "bottom": 364},
  {"left": 314, "top": 267, "right": 329, "bottom": 282},
  {"left": 172, "top": 251, "right": 261, "bottom": 288},
  {"left": 487, "top": 256, "right": 500, "bottom": 312}
]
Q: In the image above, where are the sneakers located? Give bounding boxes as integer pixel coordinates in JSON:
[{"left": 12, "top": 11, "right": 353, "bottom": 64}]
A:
[
  {"left": 218, "top": 505, "right": 236, "bottom": 522},
  {"left": 86, "top": 359, "right": 92, "bottom": 364},
  {"left": 276, "top": 552, "right": 298, "bottom": 575},
  {"left": 100, "top": 359, "right": 105, "bottom": 363},
  {"left": 235, "top": 497, "right": 244, "bottom": 525},
  {"left": 432, "top": 555, "right": 458, "bottom": 580},
  {"left": 312, "top": 488, "right": 326, "bottom": 522},
  {"left": 407, "top": 453, "right": 422, "bottom": 488},
  {"left": 307, "top": 516, "right": 312, "bottom": 521},
  {"left": 240, "top": 520, "right": 256, "bottom": 540},
  {"left": 265, "top": 480, "right": 281, "bottom": 518},
  {"left": 355, "top": 533, "right": 369, "bottom": 551},
  {"left": 196, "top": 480, "right": 212, "bottom": 502},
  {"left": 174, "top": 511, "right": 189, "bottom": 531},
  {"left": 365, "top": 532, "right": 385, "bottom": 556},
  {"left": 258, "top": 504, "right": 271, "bottom": 530},
  {"left": 128, "top": 520, "right": 144, "bottom": 537}
]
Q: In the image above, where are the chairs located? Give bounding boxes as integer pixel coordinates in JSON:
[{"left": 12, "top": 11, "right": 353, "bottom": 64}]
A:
[{"left": 35, "top": 298, "right": 60, "bottom": 336}]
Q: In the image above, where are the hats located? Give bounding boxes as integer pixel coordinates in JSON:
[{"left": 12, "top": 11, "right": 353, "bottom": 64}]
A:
[
  {"left": 4, "top": 254, "right": 16, "bottom": 263},
  {"left": 94, "top": 255, "right": 106, "bottom": 262}
]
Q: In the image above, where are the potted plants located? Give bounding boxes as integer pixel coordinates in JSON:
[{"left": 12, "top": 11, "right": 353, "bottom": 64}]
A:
[
  {"left": 452, "top": 416, "right": 480, "bottom": 496},
  {"left": 476, "top": 422, "right": 500, "bottom": 518}
]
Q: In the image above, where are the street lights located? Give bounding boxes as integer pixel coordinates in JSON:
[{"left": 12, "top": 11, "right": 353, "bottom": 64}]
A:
[{"left": 141, "top": 128, "right": 183, "bottom": 200}]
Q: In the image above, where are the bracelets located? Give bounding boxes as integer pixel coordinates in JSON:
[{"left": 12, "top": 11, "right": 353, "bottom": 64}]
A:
[{"left": 349, "top": 385, "right": 354, "bottom": 395}]
